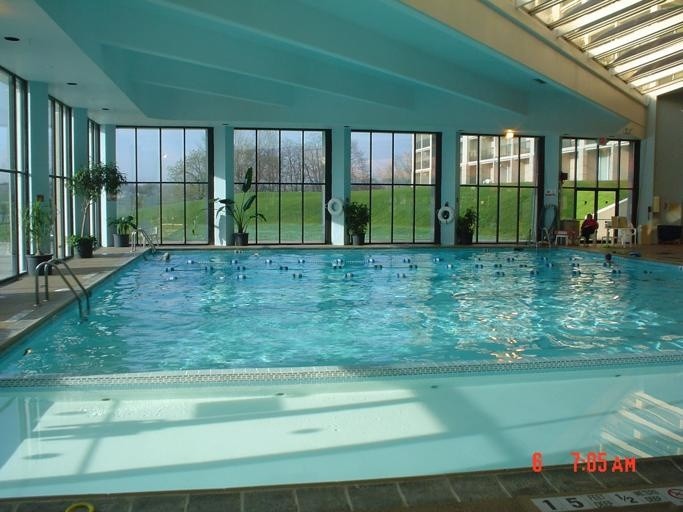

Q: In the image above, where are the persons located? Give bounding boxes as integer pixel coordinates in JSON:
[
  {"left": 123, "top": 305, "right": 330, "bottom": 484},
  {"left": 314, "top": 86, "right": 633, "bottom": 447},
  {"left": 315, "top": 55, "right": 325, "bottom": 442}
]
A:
[
  {"left": 605, "top": 253, "right": 612, "bottom": 267},
  {"left": 577, "top": 214, "right": 599, "bottom": 247}
]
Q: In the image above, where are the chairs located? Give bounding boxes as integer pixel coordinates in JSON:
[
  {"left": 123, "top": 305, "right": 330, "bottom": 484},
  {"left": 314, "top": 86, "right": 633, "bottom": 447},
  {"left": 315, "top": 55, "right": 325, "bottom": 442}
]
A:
[
  {"left": 555, "top": 224, "right": 637, "bottom": 246},
  {"left": 140, "top": 226, "right": 159, "bottom": 247}
]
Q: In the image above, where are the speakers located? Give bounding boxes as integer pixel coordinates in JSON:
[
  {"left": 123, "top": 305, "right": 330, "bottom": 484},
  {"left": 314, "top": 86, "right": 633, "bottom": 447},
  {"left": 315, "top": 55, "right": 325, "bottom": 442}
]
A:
[{"left": 561, "top": 173, "right": 567, "bottom": 180}]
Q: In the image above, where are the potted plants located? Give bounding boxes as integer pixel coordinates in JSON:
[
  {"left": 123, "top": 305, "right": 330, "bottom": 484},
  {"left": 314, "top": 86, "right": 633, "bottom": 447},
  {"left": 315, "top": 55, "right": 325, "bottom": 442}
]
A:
[
  {"left": 107, "top": 215, "right": 138, "bottom": 247},
  {"left": 69, "top": 162, "right": 128, "bottom": 258},
  {"left": 192, "top": 167, "right": 267, "bottom": 246},
  {"left": 455, "top": 206, "right": 479, "bottom": 245},
  {"left": 23, "top": 199, "right": 57, "bottom": 275},
  {"left": 343, "top": 199, "right": 371, "bottom": 245}
]
